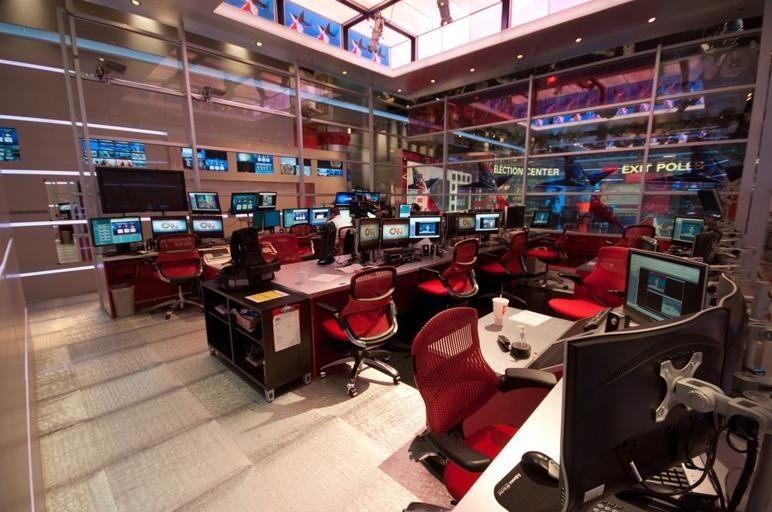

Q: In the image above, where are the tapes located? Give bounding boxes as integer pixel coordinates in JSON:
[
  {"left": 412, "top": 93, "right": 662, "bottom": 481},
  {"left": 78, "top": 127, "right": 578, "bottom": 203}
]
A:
[{"left": 511, "top": 342, "right": 532, "bottom": 359}]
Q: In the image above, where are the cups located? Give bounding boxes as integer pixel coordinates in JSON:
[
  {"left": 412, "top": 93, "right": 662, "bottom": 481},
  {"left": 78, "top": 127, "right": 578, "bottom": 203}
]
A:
[{"left": 492, "top": 298, "right": 509, "bottom": 326}]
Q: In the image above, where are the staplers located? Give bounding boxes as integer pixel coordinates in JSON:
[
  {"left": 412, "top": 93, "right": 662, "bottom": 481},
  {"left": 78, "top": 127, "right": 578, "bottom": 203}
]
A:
[{"left": 498, "top": 335, "right": 511, "bottom": 352}]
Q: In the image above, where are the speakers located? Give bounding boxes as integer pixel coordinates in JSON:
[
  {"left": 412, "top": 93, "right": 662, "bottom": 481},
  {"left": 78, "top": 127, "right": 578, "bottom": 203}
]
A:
[{"left": 606, "top": 312, "right": 630, "bottom": 334}]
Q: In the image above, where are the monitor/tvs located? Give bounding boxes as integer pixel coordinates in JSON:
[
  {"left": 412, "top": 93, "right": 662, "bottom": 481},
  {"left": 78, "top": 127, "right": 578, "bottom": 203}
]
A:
[
  {"left": 623, "top": 247, "right": 710, "bottom": 327},
  {"left": 587, "top": 220, "right": 611, "bottom": 234},
  {"left": 697, "top": 190, "right": 726, "bottom": 220},
  {"left": 81, "top": 140, "right": 553, "bottom": 268},
  {"left": 719, "top": 273, "right": 751, "bottom": 402},
  {"left": 671, "top": 216, "right": 706, "bottom": 249},
  {"left": 641, "top": 235, "right": 660, "bottom": 253},
  {"left": 1, "top": 127, "right": 22, "bottom": 164},
  {"left": 562, "top": 312, "right": 730, "bottom": 512}
]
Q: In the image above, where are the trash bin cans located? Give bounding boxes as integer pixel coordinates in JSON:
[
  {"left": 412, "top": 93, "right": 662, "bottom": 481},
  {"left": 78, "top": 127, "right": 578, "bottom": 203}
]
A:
[{"left": 110, "top": 282, "right": 136, "bottom": 317}]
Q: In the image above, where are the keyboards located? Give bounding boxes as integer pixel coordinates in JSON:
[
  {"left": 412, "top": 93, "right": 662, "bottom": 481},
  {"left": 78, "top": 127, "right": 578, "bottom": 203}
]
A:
[{"left": 636, "top": 464, "right": 692, "bottom": 495}]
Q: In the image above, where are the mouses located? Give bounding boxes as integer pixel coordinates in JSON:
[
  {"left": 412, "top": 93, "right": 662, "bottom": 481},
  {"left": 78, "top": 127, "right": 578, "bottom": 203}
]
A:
[{"left": 522, "top": 452, "right": 562, "bottom": 488}]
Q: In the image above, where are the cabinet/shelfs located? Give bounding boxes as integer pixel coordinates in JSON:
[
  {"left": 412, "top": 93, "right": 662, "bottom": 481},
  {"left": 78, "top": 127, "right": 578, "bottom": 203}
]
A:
[{"left": 200, "top": 277, "right": 312, "bottom": 402}]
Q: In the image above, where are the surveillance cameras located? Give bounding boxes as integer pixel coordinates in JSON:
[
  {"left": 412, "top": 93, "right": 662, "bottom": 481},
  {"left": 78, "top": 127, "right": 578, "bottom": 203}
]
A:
[
  {"left": 98, "top": 57, "right": 127, "bottom": 76},
  {"left": 304, "top": 104, "right": 321, "bottom": 115},
  {"left": 204, "top": 86, "right": 227, "bottom": 97}
]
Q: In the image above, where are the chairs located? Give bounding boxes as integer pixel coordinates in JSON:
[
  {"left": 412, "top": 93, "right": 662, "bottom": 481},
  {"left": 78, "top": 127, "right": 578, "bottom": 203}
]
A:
[
  {"left": 262, "top": 222, "right": 356, "bottom": 266},
  {"left": 481, "top": 224, "right": 660, "bottom": 319},
  {"left": 412, "top": 307, "right": 555, "bottom": 503},
  {"left": 316, "top": 239, "right": 481, "bottom": 396},
  {"left": 145, "top": 233, "right": 203, "bottom": 319}
]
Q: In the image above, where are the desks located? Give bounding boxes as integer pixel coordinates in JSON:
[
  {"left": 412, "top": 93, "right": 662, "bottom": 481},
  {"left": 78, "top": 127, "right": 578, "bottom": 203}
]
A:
[
  {"left": 257, "top": 243, "right": 502, "bottom": 380},
  {"left": 451, "top": 303, "right": 771, "bottom": 512},
  {"left": 96, "top": 239, "right": 231, "bottom": 320}
]
